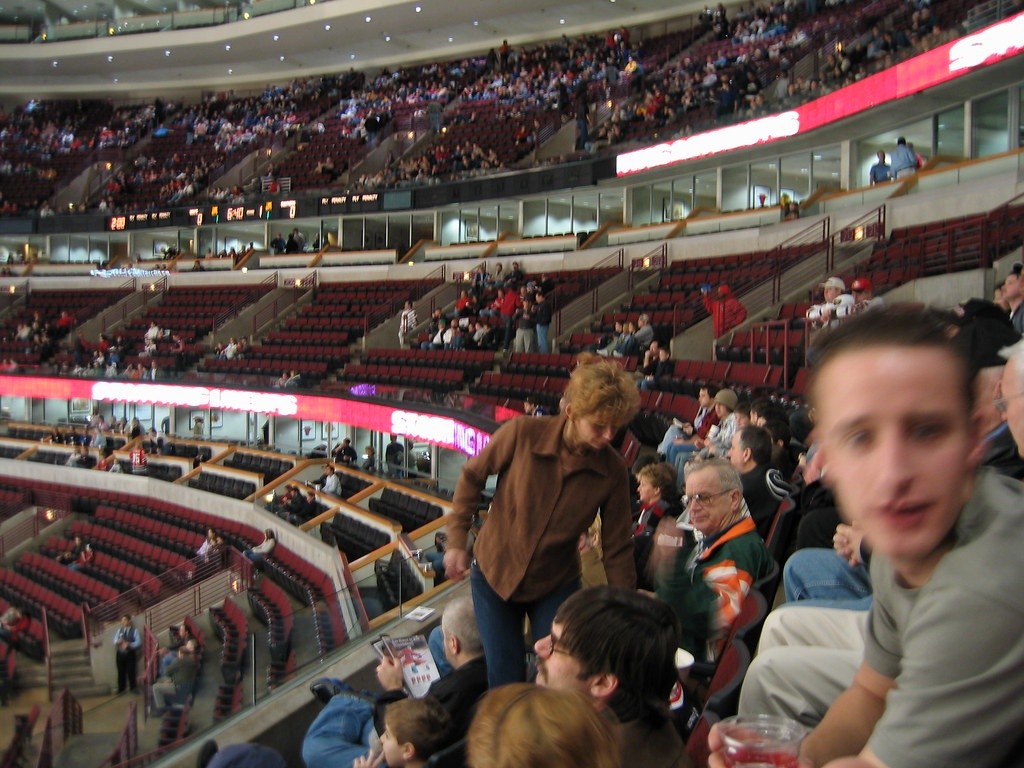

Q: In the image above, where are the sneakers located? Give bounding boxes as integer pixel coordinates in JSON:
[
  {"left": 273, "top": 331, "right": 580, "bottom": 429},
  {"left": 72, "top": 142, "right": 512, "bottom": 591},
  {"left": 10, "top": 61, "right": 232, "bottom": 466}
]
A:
[{"left": 310, "top": 678, "right": 379, "bottom": 706}]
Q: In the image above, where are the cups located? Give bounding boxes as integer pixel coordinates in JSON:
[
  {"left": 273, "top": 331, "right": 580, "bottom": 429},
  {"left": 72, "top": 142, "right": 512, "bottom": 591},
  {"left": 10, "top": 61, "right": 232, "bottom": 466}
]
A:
[
  {"left": 707, "top": 424, "right": 719, "bottom": 439},
  {"left": 718, "top": 714, "right": 806, "bottom": 768},
  {"left": 675, "top": 433, "right": 683, "bottom": 439}
]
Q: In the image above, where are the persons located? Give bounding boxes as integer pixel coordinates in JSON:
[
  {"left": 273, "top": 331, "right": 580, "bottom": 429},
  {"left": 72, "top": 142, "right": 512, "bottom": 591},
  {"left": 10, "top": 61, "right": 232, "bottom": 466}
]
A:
[
  {"left": 0, "top": 308, "right": 185, "bottom": 382},
  {"left": 269, "top": 228, "right": 320, "bottom": 254},
  {"left": 204, "top": 240, "right": 253, "bottom": 262},
  {"left": 0, "top": 606, "right": 30, "bottom": 641},
  {"left": 0, "top": 0, "right": 1024, "bottom": 214},
  {"left": 113, "top": 615, "right": 141, "bottom": 693},
  {"left": 149, "top": 625, "right": 199, "bottom": 717},
  {"left": 57, "top": 535, "right": 95, "bottom": 570},
  {"left": 151, "top": 264, "right": 167, "bottom": 271},
  {"left": 0, "top": 250, "right": 31, "bottom": 277},
  {"left": 162, "top": 247, "right": 180, "bottom": 260},
  {"left": 195, "top": 529, "right": 225, "bottom": 568},
  {"left": 192, "top": 260, "right": 205, "bottom": 272},
  {"left": 242, "top": 528, "right": 274, "bottom": 563},
  {"left": 51, "top": 413, "right": 211, "bottom": 474},
  {"left": 209, "top": 260, "right": 1024, "bottom": 768}
]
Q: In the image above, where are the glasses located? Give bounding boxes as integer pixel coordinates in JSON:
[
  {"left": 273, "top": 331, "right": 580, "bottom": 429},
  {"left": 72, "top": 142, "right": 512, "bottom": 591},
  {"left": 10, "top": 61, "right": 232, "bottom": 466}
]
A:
[
  {"left": 681, "top": 489, "right": 733, "bottom": 505},
  {"left": 548, "top": 633, "right": 573, "bottom": 657}
]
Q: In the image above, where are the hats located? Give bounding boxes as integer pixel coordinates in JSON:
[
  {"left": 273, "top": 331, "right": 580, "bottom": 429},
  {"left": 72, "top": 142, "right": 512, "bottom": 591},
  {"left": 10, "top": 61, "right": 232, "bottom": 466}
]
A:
[
  {"left": 850, "top": 278, "right": 871, "bottom": 290},
  {"left": 819, "top": 277, "right": 845, "bottom": 290},
  {"left": 930, "top": 297, "right": 1010, "bottom": 331},
  {"left": 714, "top": 389, "right": 738, "bottom": 411}
]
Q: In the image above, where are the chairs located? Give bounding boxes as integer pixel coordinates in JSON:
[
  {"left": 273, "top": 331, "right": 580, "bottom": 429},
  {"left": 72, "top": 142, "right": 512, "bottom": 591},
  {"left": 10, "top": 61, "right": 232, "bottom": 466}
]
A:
[{"left": 0, "top": 0, "right": 1024, "bottom": 768}]
